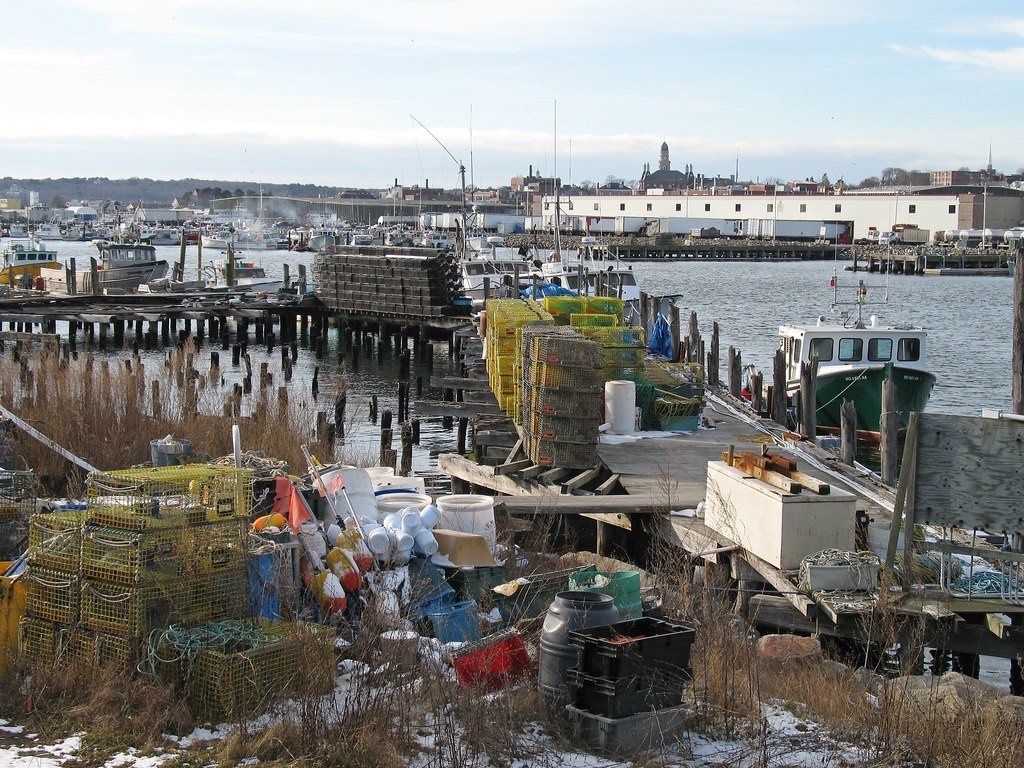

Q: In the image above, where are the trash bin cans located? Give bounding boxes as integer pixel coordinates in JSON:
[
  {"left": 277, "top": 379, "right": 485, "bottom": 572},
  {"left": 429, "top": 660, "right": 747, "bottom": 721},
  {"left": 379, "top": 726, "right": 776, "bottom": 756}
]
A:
[
  {"left": 453, "top": 633, "right": 533, "bottom": 696},
  {"left": 568, "top": 570, "right": 643, "bottom": 622}
]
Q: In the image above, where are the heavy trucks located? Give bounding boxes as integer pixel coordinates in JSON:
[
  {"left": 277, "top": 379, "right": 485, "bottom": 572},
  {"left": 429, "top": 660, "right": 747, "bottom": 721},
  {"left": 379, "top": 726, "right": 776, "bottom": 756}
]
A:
[{"left": 879, "top": 224, "right": 931, "bottom": 247}]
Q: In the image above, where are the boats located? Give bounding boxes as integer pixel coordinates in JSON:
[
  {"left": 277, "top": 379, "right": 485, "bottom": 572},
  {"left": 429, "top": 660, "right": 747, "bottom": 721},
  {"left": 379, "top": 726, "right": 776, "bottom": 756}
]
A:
[
  {"left": 41, "top": 221, "right": 170, "bottom": 294},
  {"left": 0, "top": 208, "right": 63, "bottom": 287},
  {"left": 0, "top": 208, "right": 447, "bottom": 251},
  {"left": 203, "top": 239, "right": 312, "bottom": 295},
  {"left": 533, "top": 97, "right": 684, "bottom": 343},
  {"left": 756, "top": 218, "right": 937, "bottom": 444},
  {"left": 447, "top": 145, "right": 539, "bottom": 301}
]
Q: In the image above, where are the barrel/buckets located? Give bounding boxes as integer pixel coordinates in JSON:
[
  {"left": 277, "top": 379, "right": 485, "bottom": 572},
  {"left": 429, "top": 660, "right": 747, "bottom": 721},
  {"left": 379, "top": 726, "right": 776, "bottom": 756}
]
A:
[
  {"left": 295, "top": 463, "right": 496, "bottom": 643},
  {"left": 150, "top": 438, "right": 191, "bottom": 467},
  {"left": 569, "top": 570, "right": 642, "bottom": 621},
  {"left": 537, "top": 590, "right": 620, "bottom": 701}
]
